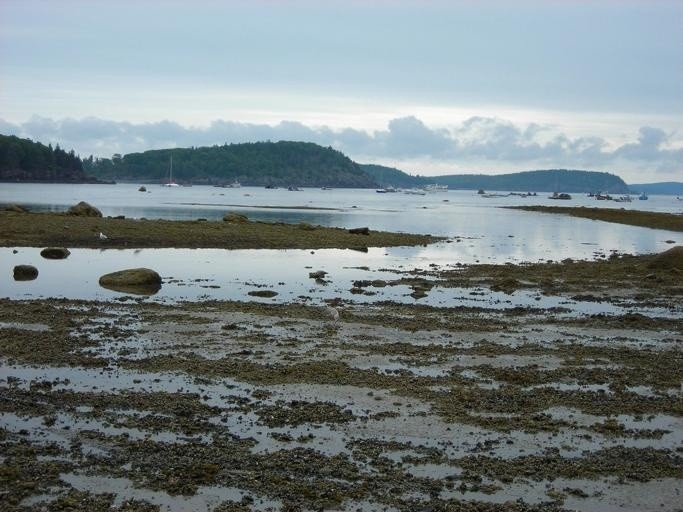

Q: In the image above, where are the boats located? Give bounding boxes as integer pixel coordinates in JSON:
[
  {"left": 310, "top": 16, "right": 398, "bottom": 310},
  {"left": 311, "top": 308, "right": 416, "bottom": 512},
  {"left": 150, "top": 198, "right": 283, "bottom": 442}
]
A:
[
  {"left": 546, "top": 190, "right": 648, "bottom": 202},
  {"left": 374, "top": 181, "right": 450, "bottom": 195},
  {"left": 218, "top": 179, "right": 331, "bottom": 191}
]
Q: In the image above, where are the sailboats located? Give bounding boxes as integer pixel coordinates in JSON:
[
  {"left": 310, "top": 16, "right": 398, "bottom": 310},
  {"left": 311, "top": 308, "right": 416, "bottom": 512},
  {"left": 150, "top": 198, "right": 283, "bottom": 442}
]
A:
[{"left": 163, "top": 151, "right": 180, "bottom": 188}]
